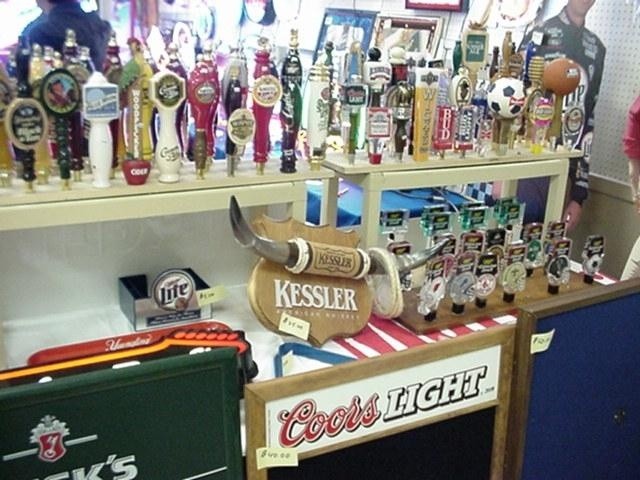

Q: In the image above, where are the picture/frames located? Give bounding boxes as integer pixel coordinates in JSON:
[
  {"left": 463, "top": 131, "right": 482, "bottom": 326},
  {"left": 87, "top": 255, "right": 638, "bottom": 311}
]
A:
[
  {"left": 0, "top": 345, "right": 246, "bottom": 480},
  {"left": 243, "top": 324, "right": 512, "bottom": 479},
  {"left": 508, "top": 277, "right": 640, "bottom": 480}
]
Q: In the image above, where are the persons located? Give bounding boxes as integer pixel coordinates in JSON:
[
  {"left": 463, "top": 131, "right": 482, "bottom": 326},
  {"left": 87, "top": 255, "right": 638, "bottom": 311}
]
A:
[
  {"left": 517, "top": 1, "right": 606, "bottom": 233},
  {"left": 620, "top": 94, "right": 640, "bottom": 280},
  {"left": 18, "top": 0, "right": 111, "bottom": 165}
]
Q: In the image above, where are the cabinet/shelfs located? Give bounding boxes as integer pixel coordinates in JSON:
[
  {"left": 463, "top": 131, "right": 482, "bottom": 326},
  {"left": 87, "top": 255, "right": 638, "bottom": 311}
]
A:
[{"left": 1, "top": 145, "right": 620, "bottom": 461}]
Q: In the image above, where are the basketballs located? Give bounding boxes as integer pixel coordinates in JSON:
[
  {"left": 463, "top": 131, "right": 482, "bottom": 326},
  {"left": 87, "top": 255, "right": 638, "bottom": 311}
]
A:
[{"left": 540, "top": 57, "right": 580, "bottom": 94}]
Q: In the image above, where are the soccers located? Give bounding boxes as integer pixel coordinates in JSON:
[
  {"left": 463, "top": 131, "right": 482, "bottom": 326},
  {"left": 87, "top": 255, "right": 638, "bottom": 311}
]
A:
[{"left": 486, "top": 78, "right": 528, "bottom": 118}]
